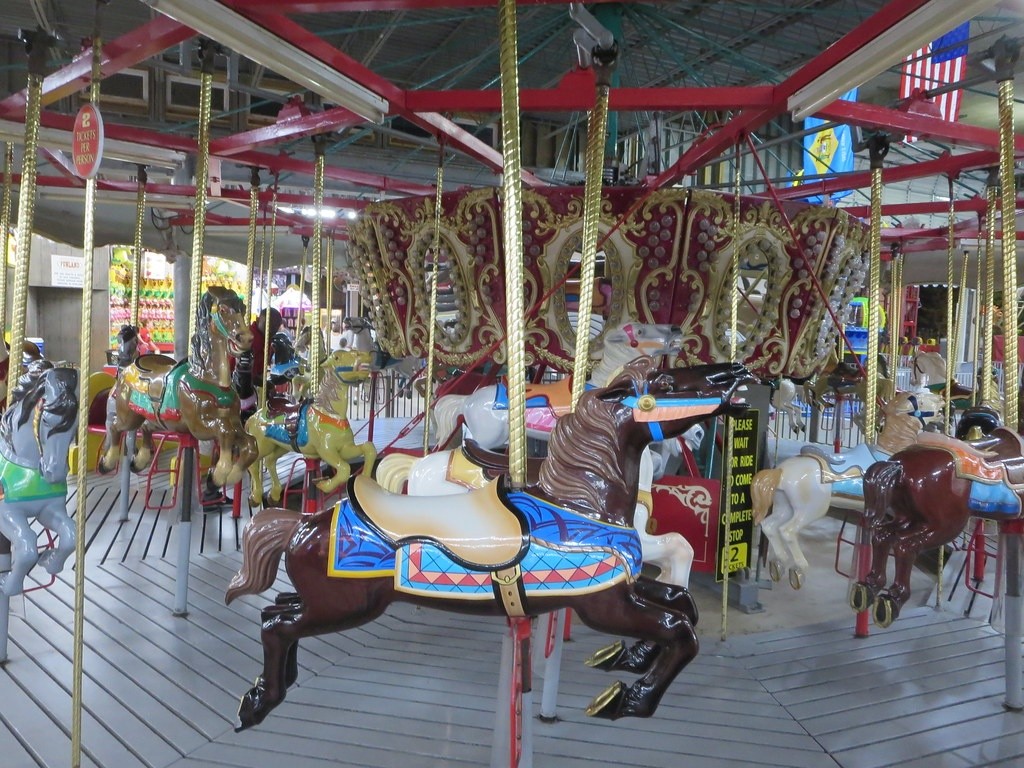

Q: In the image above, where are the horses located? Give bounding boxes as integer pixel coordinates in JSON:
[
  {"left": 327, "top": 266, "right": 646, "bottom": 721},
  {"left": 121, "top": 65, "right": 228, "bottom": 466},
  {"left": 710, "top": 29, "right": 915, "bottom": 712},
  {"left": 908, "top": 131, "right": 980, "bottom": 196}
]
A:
[
  {"left": 0, "top": 286, "right": 375, "bottom": 597},
  {"left": 225, "top": 357, "right": 1024, "bottom": 734}
]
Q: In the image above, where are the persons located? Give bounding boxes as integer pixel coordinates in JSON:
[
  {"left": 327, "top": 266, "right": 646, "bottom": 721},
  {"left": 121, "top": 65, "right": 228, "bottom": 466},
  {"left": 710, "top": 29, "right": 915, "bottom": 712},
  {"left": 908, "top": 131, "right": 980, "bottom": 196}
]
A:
[
  {"left": 200, "top": 307, "right": 282, "bottom": 515},
  {"left": 324, "top": 320, "right": 355, "bottom": 353}
]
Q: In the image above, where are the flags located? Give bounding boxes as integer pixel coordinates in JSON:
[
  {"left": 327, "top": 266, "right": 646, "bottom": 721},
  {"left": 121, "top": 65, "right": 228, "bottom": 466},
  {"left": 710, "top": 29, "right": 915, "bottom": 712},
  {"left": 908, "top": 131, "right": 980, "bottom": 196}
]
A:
[
  {"left": 804, "top": 90, "right": 856, "bottom": 203},
  {"left": 900, "top": 21, "right": 970, "bottom": 143}
]
